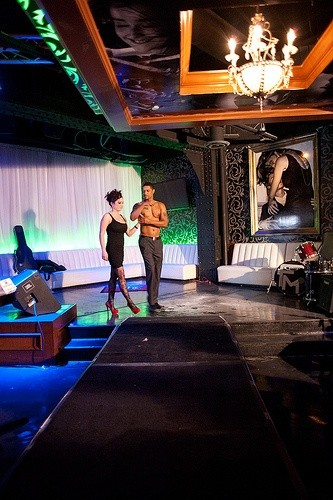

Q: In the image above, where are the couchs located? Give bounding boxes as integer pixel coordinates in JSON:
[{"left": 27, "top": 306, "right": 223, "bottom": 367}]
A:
[
  {"left": 140, "top": 245, "right": 199, "bottom": 280},
  {"left": 0, "top": 246, "right": 142, "bottom": 296},
  {"left": 217, "top": 242, "right": 323, "bottom": 285}
]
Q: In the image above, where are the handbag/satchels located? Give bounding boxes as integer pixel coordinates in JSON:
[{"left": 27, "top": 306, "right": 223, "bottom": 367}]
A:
[{"left": 36, "top": 259, "right": 57, "bottom": 281}]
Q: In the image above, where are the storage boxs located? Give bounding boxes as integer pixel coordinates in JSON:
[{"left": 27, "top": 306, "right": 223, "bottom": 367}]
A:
[{"left": 0, "top": 303, "right": 77, "bottom": 364}]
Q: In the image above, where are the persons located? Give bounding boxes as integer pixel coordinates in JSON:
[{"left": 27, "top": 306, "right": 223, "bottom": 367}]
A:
[
  {"left": 100, "top": 188, "right": 140, "bottom": 314},
  {"left": 256, "top": 147, "right": 316, "bottom": 228},
  {"left": 130, "top": 182, "right": 168, "bottom": 309},
  {"left": 98, "top": 0, "right": 236, "bottom": 114}
]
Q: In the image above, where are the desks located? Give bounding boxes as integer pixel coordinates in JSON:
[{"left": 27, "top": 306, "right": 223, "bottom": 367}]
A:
[{"left": 304, "top": 271, "right": 333, "bottom": 313}]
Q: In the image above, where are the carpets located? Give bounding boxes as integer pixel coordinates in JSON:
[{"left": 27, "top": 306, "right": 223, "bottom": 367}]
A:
[{"left": 0, "top": 316, "right": 312, "bottom": 500}]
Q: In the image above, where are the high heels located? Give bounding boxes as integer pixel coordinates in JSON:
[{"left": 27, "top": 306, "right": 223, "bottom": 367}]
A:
[
  {"left": 127, "top": 303, "right": 140, "bottom": 314},
  {"left": 106, "top": 301, "right": 118, "bottom": 314}
]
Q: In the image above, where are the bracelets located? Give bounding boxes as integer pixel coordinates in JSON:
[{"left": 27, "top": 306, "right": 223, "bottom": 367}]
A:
[{"left": 134, "top": 226, "right": 138, "bottom": 229}]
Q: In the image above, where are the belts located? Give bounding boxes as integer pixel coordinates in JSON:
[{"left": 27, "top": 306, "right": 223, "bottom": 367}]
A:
[{"left": 141, "top": 236, "right": 161, "bottom": 240}]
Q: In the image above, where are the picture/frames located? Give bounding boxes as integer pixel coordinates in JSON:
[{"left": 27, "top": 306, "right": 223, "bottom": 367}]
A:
[{"left": 247, "top": 132, "right": 320, "bottom": 236}]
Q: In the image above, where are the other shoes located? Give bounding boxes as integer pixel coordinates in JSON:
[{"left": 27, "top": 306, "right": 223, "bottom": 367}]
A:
[
  {"left": 149, "top": 305, "right": 159, "bottom": 311},
  {"left": 155, "top": 303, "right": 164, "bottom": 309}
]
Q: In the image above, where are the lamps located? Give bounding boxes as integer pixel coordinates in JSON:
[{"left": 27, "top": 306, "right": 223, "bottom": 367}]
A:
[
  {"left": 224, "top": 5, "right": 299, "bottom": 114},
  {"left": 206, "top": 126, "right": 230, "bottom": 149}
]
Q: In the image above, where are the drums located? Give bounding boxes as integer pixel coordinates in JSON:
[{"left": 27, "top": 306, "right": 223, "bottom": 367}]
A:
[
  {"left": 274, "top": 261, "right": 309, "bottom": 296},
  {"left": 295, "top": 241, "right": 319, "bottom": 262},
  {"left": 307, "top": 260, "right": 333, "bottom": 274}
]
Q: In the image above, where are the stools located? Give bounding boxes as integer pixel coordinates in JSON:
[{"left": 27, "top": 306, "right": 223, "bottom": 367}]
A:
[{"left": 276, "top": 260, "right": 305, "bottom": 294}]
